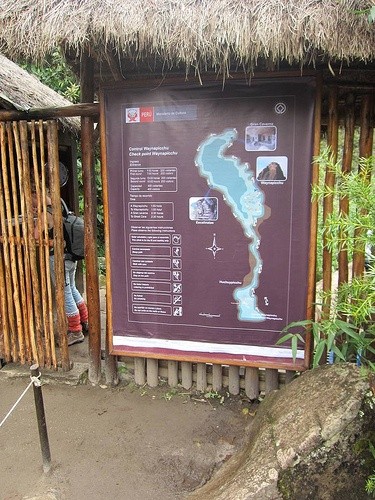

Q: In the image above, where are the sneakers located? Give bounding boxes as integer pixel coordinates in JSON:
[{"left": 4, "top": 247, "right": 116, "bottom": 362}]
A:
[{"left": 67, "top": 323, "right": 88, "bottom": 346}]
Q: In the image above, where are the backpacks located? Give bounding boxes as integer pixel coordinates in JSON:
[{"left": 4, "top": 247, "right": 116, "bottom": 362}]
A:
[{"left": 47, "top": 198, "right": 85, "bottom": 259}]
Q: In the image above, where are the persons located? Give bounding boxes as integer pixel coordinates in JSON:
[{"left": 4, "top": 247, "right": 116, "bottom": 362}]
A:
[{"left": 23, "top": 179, "right": 90, "bottom": 347}]
